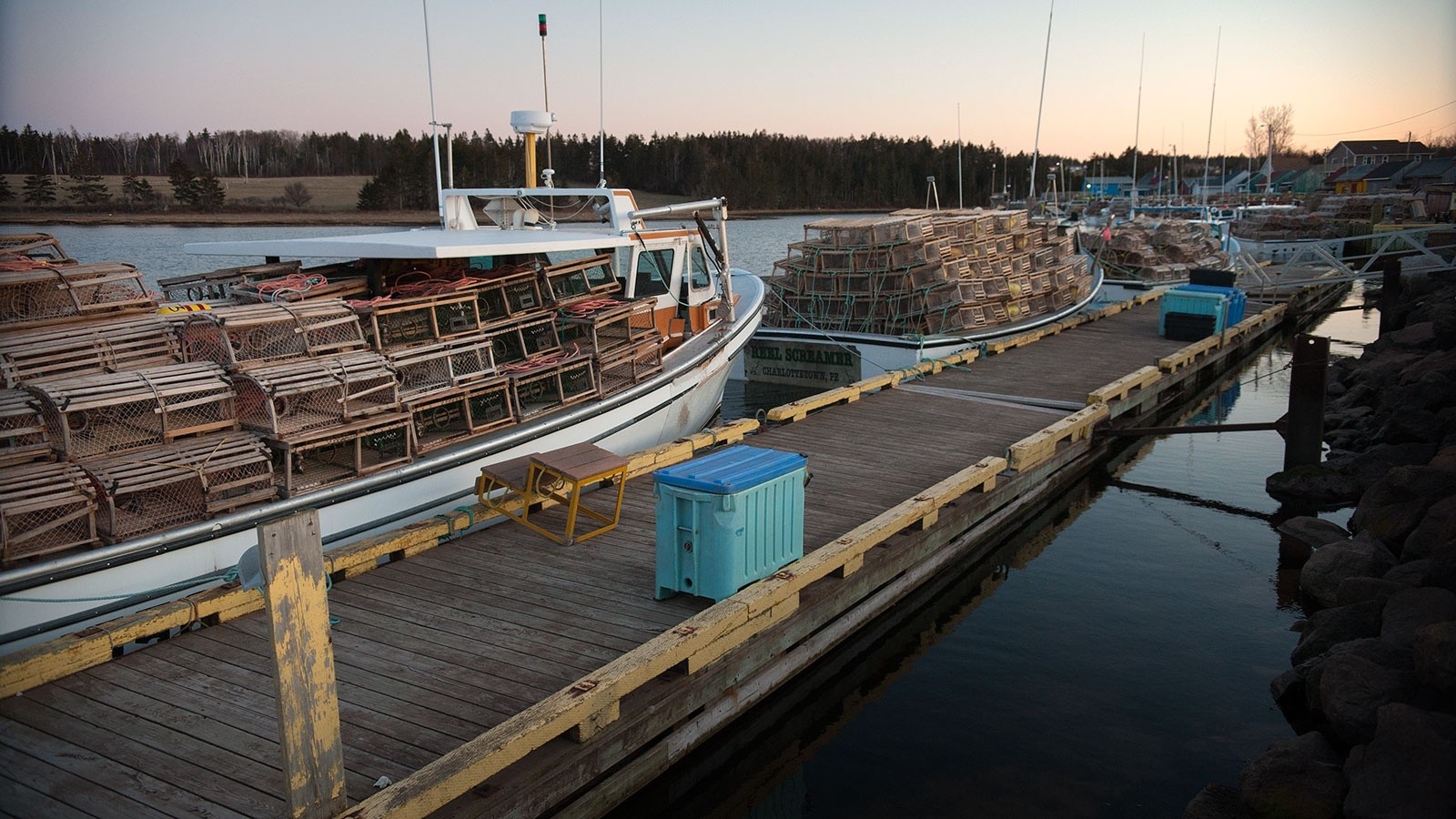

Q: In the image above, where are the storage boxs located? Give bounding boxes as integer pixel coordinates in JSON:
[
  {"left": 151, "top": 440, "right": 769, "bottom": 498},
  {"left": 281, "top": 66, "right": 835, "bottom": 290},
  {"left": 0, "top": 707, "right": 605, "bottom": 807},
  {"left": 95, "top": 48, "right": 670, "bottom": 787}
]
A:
[
  {"left": 1158, "top": 269, "right": 1247, "bottom": 341},
  {"left": 651, "top": 444, "right": 810, "bottom": 604}
]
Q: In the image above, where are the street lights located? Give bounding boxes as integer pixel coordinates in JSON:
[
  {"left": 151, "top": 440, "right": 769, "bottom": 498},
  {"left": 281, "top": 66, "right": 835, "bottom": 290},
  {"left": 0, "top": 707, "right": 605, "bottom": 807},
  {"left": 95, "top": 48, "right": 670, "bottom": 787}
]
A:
[
  {"left": 1056, "top": 159, "right": 1065, "bottom": 201},
  {"left": 1168, "top": 144, "right": 1178, "bottom": 199},
  {"left": 1260, "top": 124, "right": 1271, "bottom": 195}
]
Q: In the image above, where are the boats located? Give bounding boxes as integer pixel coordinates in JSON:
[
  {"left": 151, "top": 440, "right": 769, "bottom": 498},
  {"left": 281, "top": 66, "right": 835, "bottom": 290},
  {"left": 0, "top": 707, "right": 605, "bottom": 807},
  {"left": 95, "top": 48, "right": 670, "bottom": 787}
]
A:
[
  {"left": 1, "top": 1, "right": 768, "bottom": 663},
  {"left": 742, "top": 0, "right": 1105, "bottom": 392},
  {"left": 952, "top": 1, "right": 1346, "bottom": 304}
]
